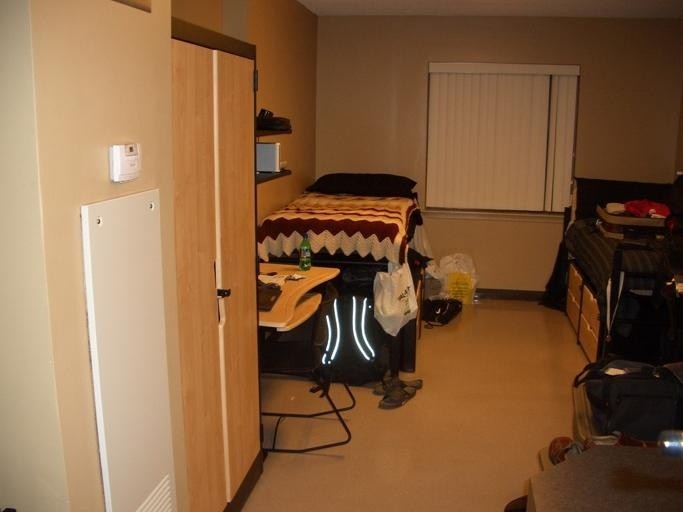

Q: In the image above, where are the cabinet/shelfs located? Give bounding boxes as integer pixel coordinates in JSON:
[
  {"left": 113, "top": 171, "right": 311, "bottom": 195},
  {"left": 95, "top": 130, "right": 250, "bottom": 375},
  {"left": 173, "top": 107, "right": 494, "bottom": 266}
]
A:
[
  {"left": 172, "top": 15, "right": 263, "bottom": 512},
  {"left": 257, "top": 124, "right": 293, "bottom": 186}
]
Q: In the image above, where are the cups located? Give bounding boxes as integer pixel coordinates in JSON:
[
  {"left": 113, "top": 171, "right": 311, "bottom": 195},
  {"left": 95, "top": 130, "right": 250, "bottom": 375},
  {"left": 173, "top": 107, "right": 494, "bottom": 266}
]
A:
[{"left": 257, "top": 108, "right": 273, "bottom": 121}]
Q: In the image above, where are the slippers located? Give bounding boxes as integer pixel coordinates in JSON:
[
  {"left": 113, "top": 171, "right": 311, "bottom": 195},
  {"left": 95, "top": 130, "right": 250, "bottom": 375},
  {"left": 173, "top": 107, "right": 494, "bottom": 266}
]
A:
[
  {"left": 374, "top": 378, "right": 422, "bottom": 395},
  {"left": 379, "top": 387, "right": 415, "bottom": 409}
]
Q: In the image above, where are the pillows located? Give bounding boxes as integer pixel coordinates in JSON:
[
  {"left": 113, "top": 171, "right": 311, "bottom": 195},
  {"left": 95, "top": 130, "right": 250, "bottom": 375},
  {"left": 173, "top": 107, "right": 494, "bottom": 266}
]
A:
[{"left": 303, "top": 173, "right": 417, "bottom": 197}]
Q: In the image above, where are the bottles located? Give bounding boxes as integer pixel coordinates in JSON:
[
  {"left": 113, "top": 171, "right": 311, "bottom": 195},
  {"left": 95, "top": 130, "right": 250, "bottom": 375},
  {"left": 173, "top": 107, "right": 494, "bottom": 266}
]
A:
[{"left": 298, "top": 234, "right": 311, "bottom": 271}]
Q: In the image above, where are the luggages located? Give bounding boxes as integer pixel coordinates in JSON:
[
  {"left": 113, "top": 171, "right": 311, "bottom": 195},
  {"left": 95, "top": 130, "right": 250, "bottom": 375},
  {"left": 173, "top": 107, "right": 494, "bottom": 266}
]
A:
[{"left": 594, "top": 202, "right": 672, "bottom": 246}]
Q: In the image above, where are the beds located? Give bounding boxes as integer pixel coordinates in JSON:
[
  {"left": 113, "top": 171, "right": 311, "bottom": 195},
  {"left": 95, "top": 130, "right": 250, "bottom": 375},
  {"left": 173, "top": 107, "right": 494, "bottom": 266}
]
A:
[
  {"left": 258, "top": 173, "right": 433, "bottom": 392},
  {"left": 562, "top": 173, "right": 682, "bottom": 364}
]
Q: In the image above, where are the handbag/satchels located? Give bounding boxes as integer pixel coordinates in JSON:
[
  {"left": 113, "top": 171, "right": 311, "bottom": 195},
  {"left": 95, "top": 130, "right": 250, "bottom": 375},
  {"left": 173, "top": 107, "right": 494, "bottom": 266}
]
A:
[
  {"left": 423, "top": 300, "right": 461, "bottom": 326},
  {"left": 572, "top": 362, "right": 681, "bottom": 441}
]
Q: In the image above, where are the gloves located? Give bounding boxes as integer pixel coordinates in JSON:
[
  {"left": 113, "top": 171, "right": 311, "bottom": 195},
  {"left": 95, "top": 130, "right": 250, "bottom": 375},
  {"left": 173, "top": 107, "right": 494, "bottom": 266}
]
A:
[{"left": 606, "top": 199, "right": 671, "bottom": 218}]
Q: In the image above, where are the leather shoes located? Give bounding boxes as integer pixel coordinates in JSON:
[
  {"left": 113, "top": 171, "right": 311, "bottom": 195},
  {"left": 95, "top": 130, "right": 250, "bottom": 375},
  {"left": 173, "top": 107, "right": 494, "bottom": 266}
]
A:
[
  {"left": 548, "top": 437, "right": 582, "bottom": 466},
  {"left": 581, "top": 431, "right": 666, "bottom": 454}
]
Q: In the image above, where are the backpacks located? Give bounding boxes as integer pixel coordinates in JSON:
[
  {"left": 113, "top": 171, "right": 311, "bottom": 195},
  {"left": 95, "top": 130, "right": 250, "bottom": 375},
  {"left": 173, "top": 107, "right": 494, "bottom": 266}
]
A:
[{"left": 311, "top": 291, "right": 375, "bottom": 385}]
